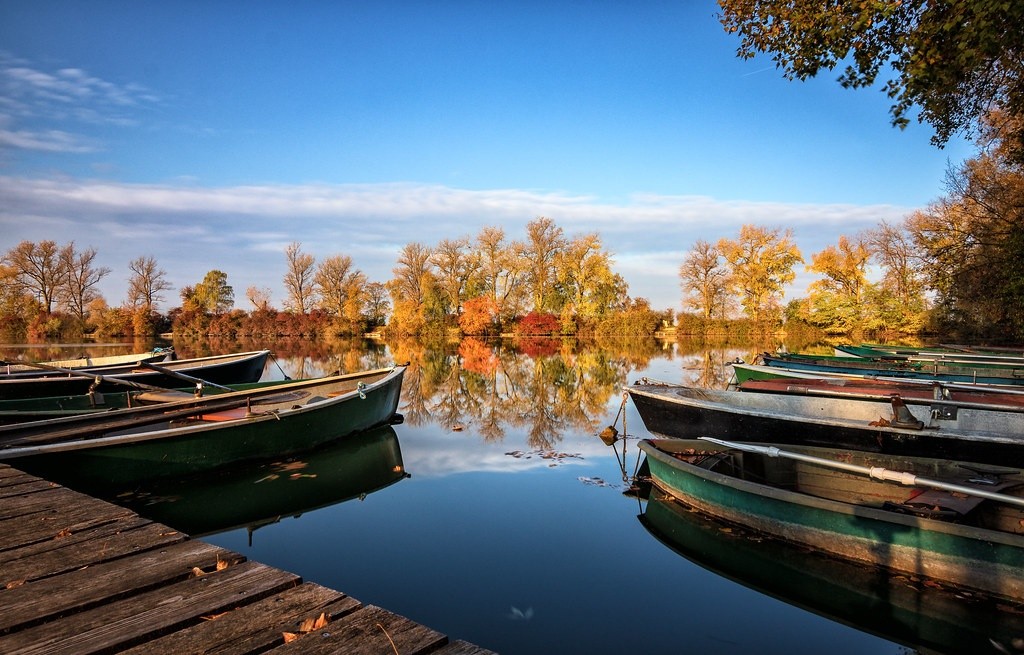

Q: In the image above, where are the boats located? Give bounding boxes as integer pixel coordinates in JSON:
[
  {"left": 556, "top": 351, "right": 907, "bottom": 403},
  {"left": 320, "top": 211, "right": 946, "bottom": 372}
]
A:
[
  {"left": 620, "top": 343, "right": 1023, "bottom": 608},
  {"left": 621, "top": 452, "right": 1024, "bottom": 655},
  {"left": 0, "top": 347, "right": 409, "bottom": 486},
  {"left": 79, "top": 421, "right": 413, "bottom": 541}
]
